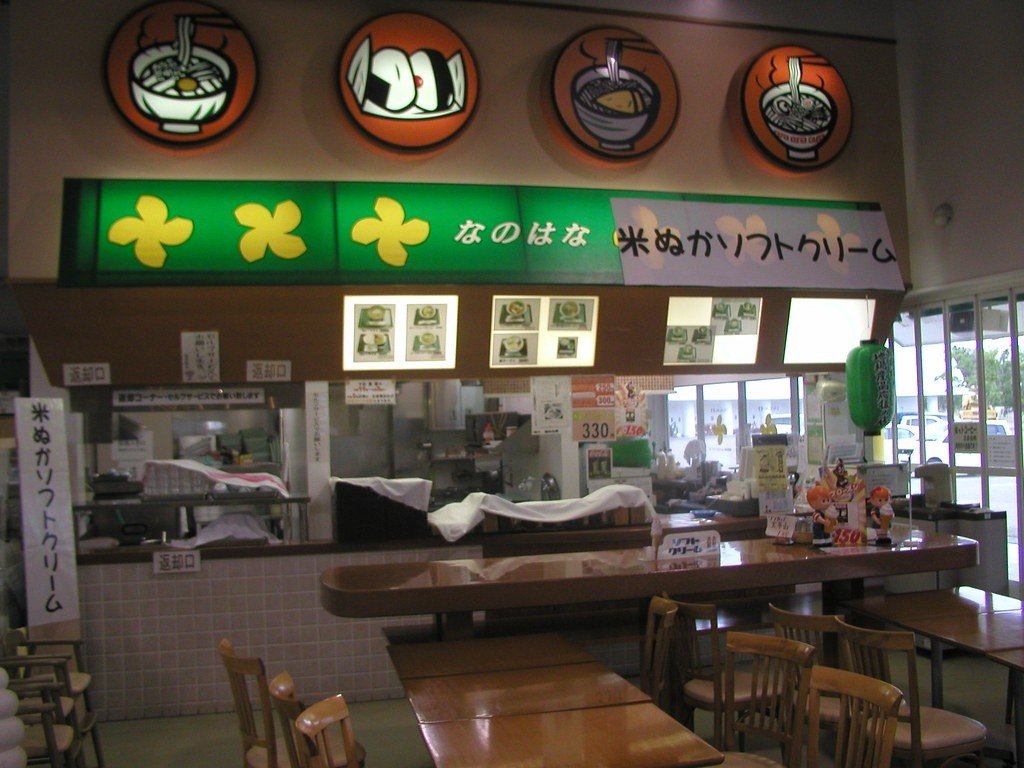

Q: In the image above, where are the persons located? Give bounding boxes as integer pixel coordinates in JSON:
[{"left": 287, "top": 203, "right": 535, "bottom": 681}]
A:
[
  {"left": 805, "top": 484, "right": 839, "bottom": 547},
  {"left": 869, "top": 485, "right": 894, "bottom": 546}
]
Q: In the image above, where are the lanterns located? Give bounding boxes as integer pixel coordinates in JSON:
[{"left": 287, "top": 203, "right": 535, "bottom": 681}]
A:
[{"left": 845, "top": 338, "right": 896, "bottom": 437}]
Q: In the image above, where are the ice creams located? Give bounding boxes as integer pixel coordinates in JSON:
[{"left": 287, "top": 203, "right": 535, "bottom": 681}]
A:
[
  {"left": 880, "top": 504, "right": 894, "bottom": 535},
  {"left": 825, "top": 503, "right": 839, "bottom": 532},
  {"left": 652, "top": 515, "right": 663, "bottom": 560}
]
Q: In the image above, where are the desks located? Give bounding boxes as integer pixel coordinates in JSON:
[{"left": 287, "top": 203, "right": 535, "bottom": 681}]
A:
[
  {"left": 846, "top": 586, "right": 1024, "bottom": 768},
  {"left": 387, "top": 631, "right": 726, "bottom": 768}
]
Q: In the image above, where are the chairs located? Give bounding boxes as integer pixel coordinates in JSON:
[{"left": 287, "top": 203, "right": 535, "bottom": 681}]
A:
[
  {"left": 835, "top": 616, "right": 988, "bottom": 768},
  {"left": 663, "top": 590, "right": 784, "bottom": 750},
  {"left": 218, "top": 638, "right": 367, "bottom": 768},
  {"left": 807, "top": 665, "right": 904, "bottom": 768},
  {"left": 0, "top": 630, "right": 106, "bottom": 768},
  {"left": 769, "top": 602, "right": 907, "bottom": 764},
  {"left": 704, "top": 630, "right": 816, "bottom": 768},
  {"left": 641, "top": 595, "right": 678, "bottom": 704}
]
